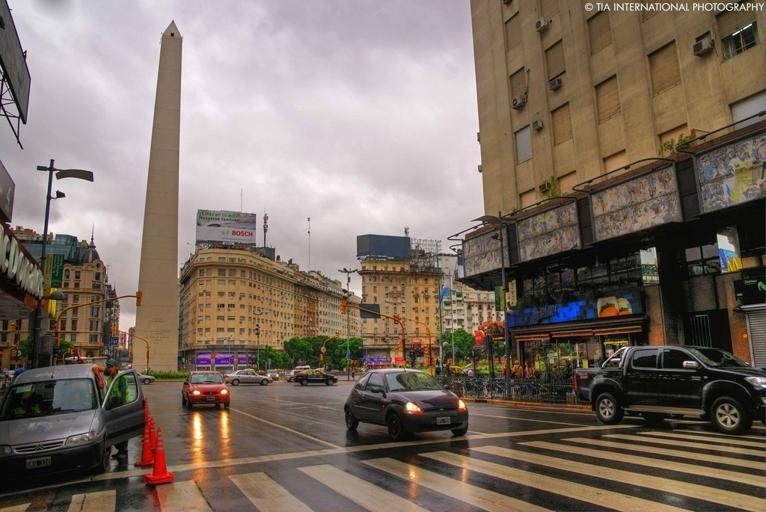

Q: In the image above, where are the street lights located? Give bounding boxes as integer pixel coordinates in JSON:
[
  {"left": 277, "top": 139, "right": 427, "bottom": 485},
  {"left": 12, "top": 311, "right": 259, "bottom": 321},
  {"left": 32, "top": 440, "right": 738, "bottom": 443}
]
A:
[
  {"left": 31, "top": 159, "right": 93, "bottom": 367},
  {"left": 475, "top": 320, "right": 504, "bottom": 377},
  {"left": 473, "top": 211, "right": 511, "bottom": 399},
  {"left": 338, "top": 268, "right": 358, "bottom": 382}
]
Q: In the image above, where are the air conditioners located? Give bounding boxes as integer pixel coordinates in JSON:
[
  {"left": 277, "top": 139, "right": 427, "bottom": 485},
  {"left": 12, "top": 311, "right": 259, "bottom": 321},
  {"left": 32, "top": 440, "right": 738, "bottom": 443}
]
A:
[
  {"left": 539, "top": 182, "right": 550, "bottom": 193},
  {"left": 692, "top": 38, "right": 712, "bottom": 56},
  {"left": 511, "top": 96, "right": 524, "bottom": 108},
  {"left": 535, "top": 17, "right": 547, "bottom": 32},
  {"left": 549, "top": 79, "right": 561, "bottom": 90},
  {"left": 533, "top": 120, "right": 543, "bottom": 130}
]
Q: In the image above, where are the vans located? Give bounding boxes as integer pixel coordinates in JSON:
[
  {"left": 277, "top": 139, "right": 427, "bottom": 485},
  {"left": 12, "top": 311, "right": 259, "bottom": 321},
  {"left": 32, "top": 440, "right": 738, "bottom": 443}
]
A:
[
  {"left": 293, "top": 365, "right": 310, "bottom": 376},
  {"left": 0, "top": 363, "right": 146, "bottom": 494}
]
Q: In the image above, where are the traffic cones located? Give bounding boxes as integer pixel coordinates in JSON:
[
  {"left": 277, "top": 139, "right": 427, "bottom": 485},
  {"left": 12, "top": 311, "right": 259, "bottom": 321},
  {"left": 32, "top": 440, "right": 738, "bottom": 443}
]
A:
[
  {"left": 144, "top": 428, "right": 174, "bottom": 485},
  {"left": 134, "top": 397, "right": 155, "bottom": 466}
]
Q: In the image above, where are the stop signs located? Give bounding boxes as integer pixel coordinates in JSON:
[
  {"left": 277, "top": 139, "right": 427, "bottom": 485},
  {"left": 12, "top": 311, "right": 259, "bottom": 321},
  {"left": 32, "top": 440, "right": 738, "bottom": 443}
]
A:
[{"left": 475, "top": 330, "right": 485, "bottom": 345}]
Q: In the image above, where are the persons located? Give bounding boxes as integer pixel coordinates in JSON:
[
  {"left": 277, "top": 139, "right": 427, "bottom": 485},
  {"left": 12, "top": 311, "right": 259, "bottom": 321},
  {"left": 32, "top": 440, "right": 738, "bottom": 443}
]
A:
[
  {"left": 5, "top": 386, "right": 44, "bottom": 419},
  {"left": 512, "top": 360, "right": 524, "bottom": 377},
  {"left": 502, "top": 364, "right": 510, "bottom": 377},
  {"left": 106, "top": 359, "right": 130, "bottom": 460},
  {"left": 523, "top": 361, "right": 535, "bottom": 378},
  {"left": 76, "top": 356, "right": 84, "bottom": 364},
  {"left": 14, "top": 363, "right": 25, "bottom": 377},
  {"left": 436, "top": 362, "right": 480, "bottom": 378}
]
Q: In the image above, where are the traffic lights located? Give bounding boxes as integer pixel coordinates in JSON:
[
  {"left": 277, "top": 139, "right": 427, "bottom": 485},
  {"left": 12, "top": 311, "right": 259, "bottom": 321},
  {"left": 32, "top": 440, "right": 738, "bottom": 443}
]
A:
[
  {"left": 394, "top": 314, "right": 399, "bottom": 323},
  {"left": 255, "top": 324, "right": 259, "bottom": 335},
  {"left": 136, "top": 293, "right": 142, "bottom": 306},
  {"left": 340, "top": 300, "right": 346, "bottom": 314},
  {"left": 112, "top": 327, "right": 116, "bottom": 337}
]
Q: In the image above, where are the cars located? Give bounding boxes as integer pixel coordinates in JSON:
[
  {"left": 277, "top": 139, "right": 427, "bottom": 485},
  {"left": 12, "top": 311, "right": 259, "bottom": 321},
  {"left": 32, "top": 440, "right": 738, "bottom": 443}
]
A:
[
  {"left": 344, "top": 368, "right": 468, "bottom": 441},
  {"left": 266, "top": 370, "right": 279, "bottom": 381},
  {"left": 119, "top": 370, "right": 155, "bottom": 385},
  {"left": 294, "top": 369, "right": 337, "bottom": 385},
  {"left": 183, "top": 371, "right": 231, "bottom": 410},
  {"left": 223, "top": 369, "right": 273, "bottom": 386}
]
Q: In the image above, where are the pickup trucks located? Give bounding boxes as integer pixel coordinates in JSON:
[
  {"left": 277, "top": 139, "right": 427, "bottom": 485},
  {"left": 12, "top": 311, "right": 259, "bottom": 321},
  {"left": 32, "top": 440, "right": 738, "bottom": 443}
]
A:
[{"left": 573, "top": 344, "right": 766, "bottom": 435}]
